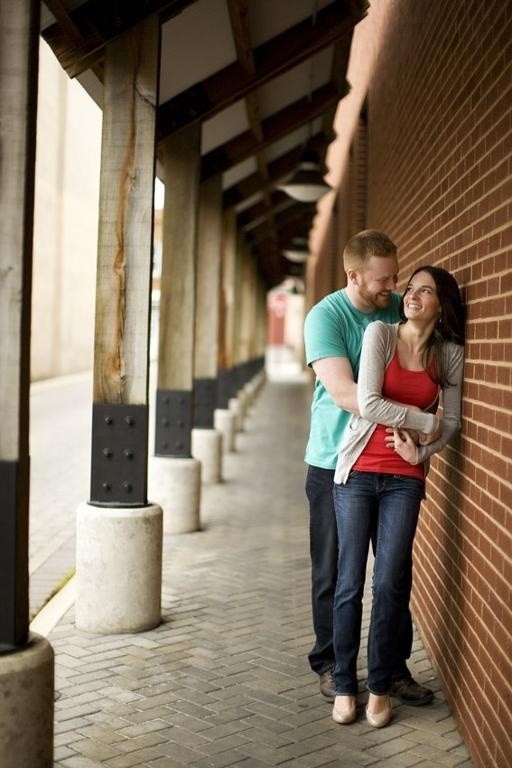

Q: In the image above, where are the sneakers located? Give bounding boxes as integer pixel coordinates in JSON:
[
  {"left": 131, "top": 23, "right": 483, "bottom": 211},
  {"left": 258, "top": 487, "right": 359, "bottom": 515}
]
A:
[
  {"left": 389, "top": 675, "right": 433, "bottom": 706},
  {"left": 320, "top": 670, "right": 335, "bottom": 703}
]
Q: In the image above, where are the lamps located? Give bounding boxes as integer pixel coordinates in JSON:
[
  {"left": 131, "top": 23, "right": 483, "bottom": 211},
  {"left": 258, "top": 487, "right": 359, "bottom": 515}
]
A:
[
  {"left": 275, "top": 66, "right": 336, "bottom": 206},
  {"left": 281, "top": 222, "right": 314, "bottom": 264}
]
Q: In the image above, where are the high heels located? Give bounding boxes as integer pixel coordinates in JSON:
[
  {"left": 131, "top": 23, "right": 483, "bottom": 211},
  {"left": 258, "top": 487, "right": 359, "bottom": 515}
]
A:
[
  {"left": 332, "top": 693, "right": 357, "bottom": 724},
  {"left": 365, "top": 690, "right": 391, "bottom": 727}
]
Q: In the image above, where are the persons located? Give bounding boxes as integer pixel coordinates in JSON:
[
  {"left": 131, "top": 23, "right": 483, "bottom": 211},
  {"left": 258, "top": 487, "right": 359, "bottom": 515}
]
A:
[
  {"left": 301, "top": 228, "right": 439, "bottom": 708},
  {"left": 326, "top": 264, "right": 465, "bottom": 724}
]
